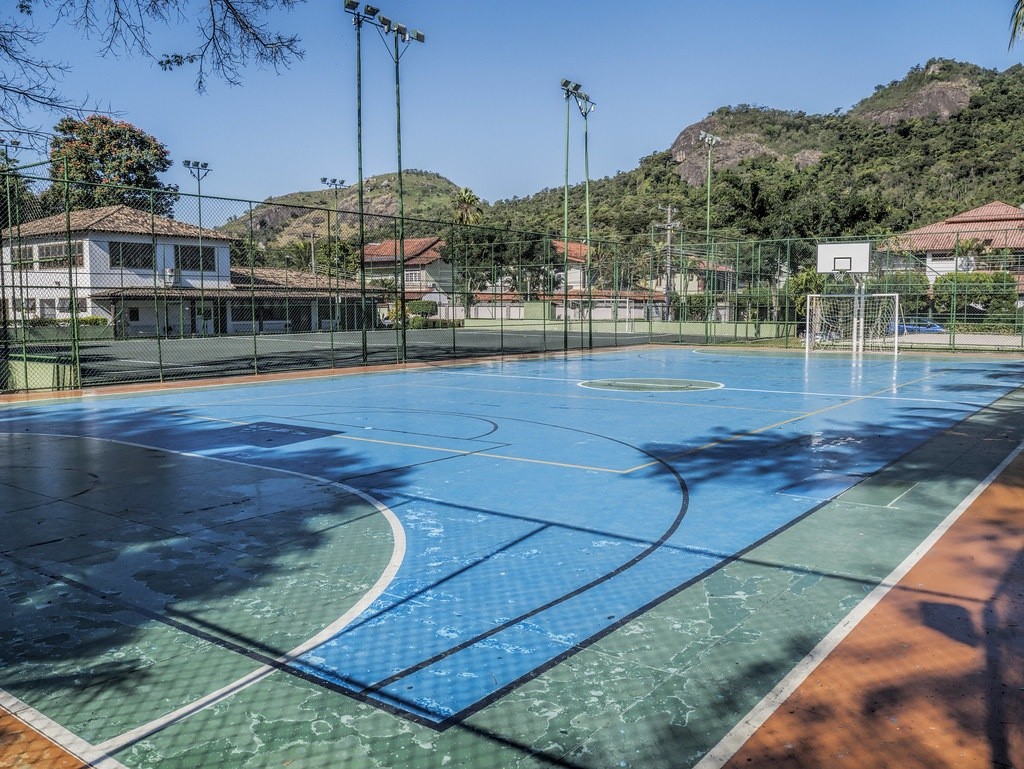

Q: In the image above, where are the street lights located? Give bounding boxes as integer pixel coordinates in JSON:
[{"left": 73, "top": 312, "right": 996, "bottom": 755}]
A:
[
  {"left": 698, "top": 131, "right": 720, "bottom": 322},
  {"left": 320, "top": 177, "right": 346, "bottom": 333},
  {"left": 342, "top": 0, "right": 380, "bottom": 363},
  {"left": 574, "top": 90, "right": 597, "bottom": 347},
  {"left": 183, "top": 160, "right": 208, "bottom": 339},
  {"left": 561, "top": 79, "right": 582, "bottom": 347},
  {"left": 376, "top": 14, "right": 425, "bottom": 366},
  {"left": 0, "top": 137, "right": 23, "bottom": 339}
]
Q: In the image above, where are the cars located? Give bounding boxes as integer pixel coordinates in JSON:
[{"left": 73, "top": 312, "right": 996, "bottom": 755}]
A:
[{"left": 885, "top": 316, "right": 945, "bottom": 336}]
